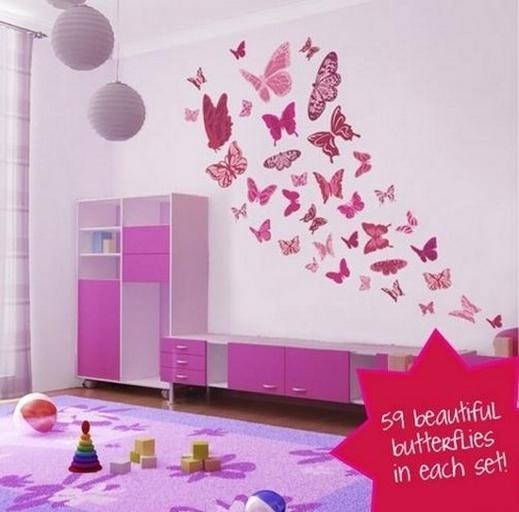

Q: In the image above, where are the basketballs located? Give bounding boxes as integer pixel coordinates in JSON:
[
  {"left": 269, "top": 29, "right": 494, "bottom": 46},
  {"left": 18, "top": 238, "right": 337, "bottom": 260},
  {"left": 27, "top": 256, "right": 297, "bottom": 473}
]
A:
[
  {"left": 245, "top": 490, "right": 286, "bottom": 512},
  {"left": 15, "top": 392, "right": 56, "bottom": 436}
]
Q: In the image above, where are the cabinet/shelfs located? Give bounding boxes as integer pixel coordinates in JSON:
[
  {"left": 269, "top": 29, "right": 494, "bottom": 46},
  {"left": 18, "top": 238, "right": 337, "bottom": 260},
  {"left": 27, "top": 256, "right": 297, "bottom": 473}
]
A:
[
  {"left": 73, "top": 194, "right": 202, "bottom": 398},
  {"left": 158, "top": 331, "right": 477, "bottom": 419}
]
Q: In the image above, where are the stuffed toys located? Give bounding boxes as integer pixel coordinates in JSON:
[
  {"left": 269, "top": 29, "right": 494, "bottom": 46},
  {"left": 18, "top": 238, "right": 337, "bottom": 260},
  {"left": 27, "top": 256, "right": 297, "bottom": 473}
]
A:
[{"left": 68, "top": 418, "right": 220, "bottom": 473}]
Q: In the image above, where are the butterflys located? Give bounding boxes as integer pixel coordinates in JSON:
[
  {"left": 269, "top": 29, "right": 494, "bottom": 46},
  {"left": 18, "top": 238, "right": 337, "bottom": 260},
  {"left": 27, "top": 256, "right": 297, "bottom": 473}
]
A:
[{"left": 183, "top": 36, "right": 503, "bottom": 329}]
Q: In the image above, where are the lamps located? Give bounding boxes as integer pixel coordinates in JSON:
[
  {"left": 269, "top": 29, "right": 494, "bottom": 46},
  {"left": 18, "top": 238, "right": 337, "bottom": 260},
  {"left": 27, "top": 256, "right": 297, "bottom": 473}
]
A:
[{"left": 53, "top": 2, "right": 147, "bottom": 143}]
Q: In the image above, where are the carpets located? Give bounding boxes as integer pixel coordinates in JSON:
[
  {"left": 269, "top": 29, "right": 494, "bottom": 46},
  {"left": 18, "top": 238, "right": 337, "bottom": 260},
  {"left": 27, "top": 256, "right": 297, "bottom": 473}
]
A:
[{"left": 1, "top": 392, "right": 372, "bottom": 512}]
